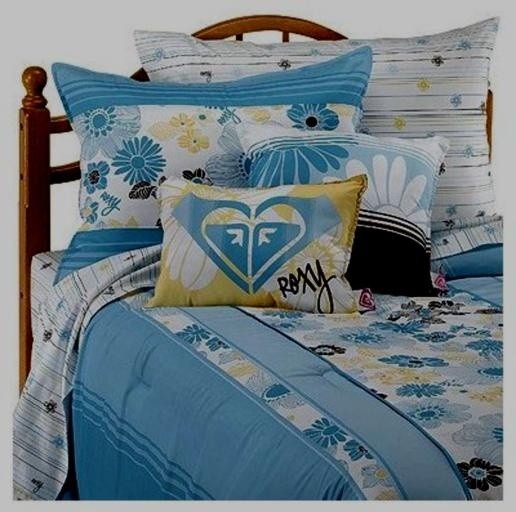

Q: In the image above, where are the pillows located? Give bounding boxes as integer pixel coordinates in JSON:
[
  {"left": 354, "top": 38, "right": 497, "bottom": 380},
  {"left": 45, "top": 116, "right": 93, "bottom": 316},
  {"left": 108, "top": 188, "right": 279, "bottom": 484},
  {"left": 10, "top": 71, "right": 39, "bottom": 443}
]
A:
[
  {"left": 234, "top": 117, "right": 448, "bottom": 297},
  {"left": 48, "top": 45, "right": 372, "bottom": 284},
  {"left": 143, "top": 174, "right": 369, "bottom": 313},
  {"left": 132, "top": 16, "right": 504, "bottom": 227}
]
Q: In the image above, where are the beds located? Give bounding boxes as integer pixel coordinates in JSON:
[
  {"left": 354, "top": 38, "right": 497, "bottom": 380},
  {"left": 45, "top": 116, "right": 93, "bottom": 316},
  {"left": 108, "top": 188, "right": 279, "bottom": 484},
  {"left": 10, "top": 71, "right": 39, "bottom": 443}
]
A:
[{"left": 9, "top": 11, "right": 503, "bottom": 500}]
